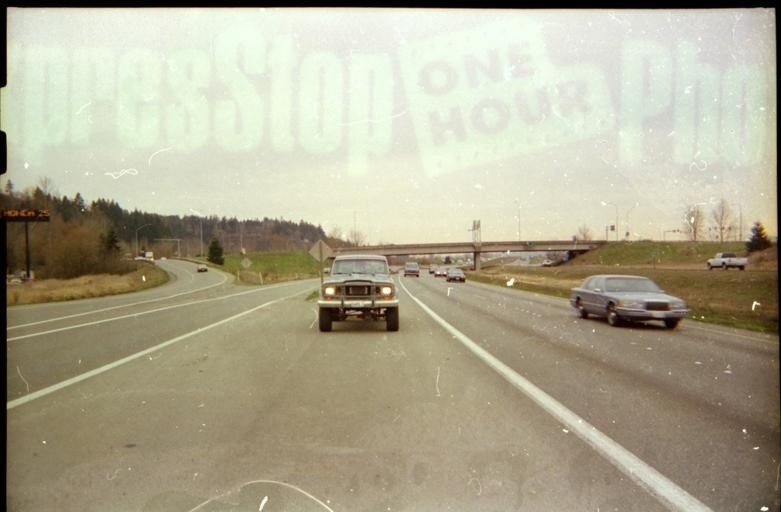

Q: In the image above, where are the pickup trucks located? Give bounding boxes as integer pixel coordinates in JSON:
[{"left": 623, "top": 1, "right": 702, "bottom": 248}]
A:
[{"left": 706, "top": 252, "right": 748, "bottom": 270}]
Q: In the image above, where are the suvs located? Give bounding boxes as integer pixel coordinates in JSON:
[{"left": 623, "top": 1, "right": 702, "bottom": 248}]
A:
[
  {"left": 404, "top": 262, "right": 420, "bottom": 277},
  {"left": 317, "top": 255, "right": 399, "bottom": 332}
]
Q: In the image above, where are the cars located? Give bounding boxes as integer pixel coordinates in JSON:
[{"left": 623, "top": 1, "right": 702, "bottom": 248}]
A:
[
  {"left": 570, "top": 274, "right": 687, "bottom": 328},
  {"left": 429, "top": 264, "right": 466, "bottom": 282},
  {"left": 197, "top": 264, "right": 208, "bottom": 272}
]
